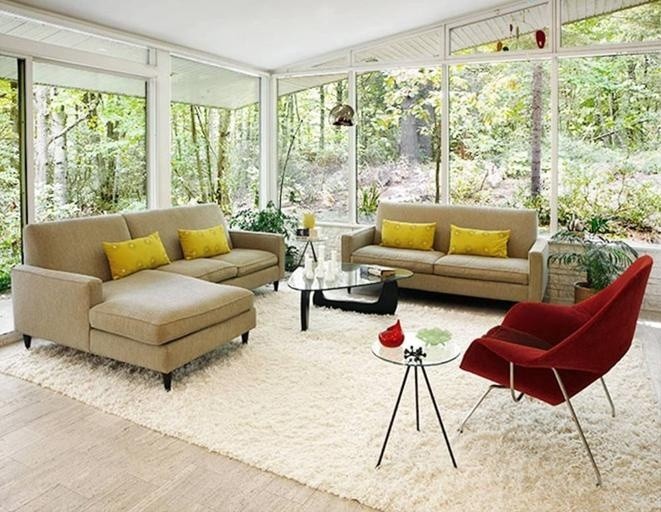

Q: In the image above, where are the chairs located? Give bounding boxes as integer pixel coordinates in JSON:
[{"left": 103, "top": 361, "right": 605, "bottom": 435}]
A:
[{"left": 451, "top": 254, "right": 660, "bottom": 490}]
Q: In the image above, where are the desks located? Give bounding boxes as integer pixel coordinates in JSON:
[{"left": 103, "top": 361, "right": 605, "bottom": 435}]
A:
[
  {"left": 369, "top": 321, "right": 467, "bottom": 476},
  {"left": 285, "top": 255, "right": 417, "bottom": 332},
  {"left": 288, "top": 231, "right": 330, "bottom": 270}
]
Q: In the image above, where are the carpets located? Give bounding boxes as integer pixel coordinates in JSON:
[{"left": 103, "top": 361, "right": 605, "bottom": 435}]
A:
[{"left": 1, "top": 261, "right": 661, "bottom": 512}]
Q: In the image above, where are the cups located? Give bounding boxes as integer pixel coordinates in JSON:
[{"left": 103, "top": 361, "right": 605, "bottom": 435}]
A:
[{"left": 310, "top": 229, "right": 317, "bottom": 238}]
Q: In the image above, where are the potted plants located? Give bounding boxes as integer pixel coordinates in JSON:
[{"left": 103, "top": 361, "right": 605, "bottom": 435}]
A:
[{"left": 533, "top": 199, "right": 660, "bottom": 309}]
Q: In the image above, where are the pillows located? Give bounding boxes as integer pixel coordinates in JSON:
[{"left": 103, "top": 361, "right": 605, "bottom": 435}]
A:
[
  {"left": 101, "top": 228, "right": 172, "bottom": 282},
  {"left": 446, "top": 220, "right": 513, "bottom": 262},
  {"left": 174, "top": 224, "right": 235, "bottom": 263},
  {"left": 379, "top": 214, "right": 438, "bottom": 254}
]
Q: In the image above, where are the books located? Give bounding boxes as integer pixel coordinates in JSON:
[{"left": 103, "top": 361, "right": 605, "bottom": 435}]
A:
[{"left": 360, "top": 264, "right": 396, "bottom": 278}]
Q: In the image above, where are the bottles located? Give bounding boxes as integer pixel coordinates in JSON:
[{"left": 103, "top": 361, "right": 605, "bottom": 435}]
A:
[{"left": 302, "top": 243, "right": 339, "bottom": 284}]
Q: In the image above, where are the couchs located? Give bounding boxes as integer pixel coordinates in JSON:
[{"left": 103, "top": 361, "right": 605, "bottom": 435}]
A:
[
  {"left": 339, "top": 201, "right": 553, "bottom": 317},
  {"left": 6, "top": 199, "right": 287, "bottom": 398}
]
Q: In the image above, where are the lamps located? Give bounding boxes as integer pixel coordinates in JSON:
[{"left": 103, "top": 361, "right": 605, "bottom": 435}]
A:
[{"left": 272, "top": 99, "right": 356, "bottom": 218}]
